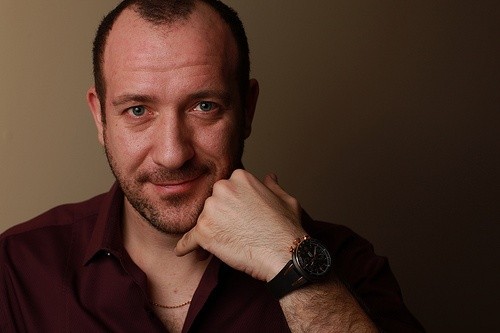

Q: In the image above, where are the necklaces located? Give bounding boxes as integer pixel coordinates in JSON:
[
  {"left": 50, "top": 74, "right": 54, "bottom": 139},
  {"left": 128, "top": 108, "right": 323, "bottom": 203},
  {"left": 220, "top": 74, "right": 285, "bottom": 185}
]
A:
[{"left": 150, "top": 298, "right": 192, "bottom": 309}]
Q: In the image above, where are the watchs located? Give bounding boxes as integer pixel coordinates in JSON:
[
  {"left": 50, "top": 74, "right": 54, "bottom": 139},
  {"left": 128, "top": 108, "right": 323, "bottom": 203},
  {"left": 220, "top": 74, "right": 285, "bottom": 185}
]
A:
[{"left": 264, "top": 235, "right": 338, "bottom": 301}]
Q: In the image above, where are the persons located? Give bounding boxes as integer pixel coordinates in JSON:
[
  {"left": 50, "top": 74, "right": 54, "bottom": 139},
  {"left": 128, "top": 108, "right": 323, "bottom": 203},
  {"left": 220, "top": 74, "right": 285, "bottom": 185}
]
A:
[{"left": 0, "top": 0, "right": 426, "bottom": 333}]
